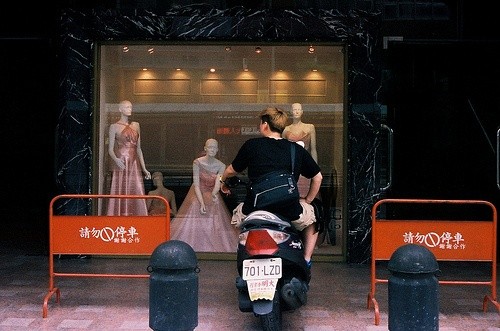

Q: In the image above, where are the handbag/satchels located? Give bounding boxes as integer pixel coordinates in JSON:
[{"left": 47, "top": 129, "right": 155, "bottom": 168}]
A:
[{"left": 249, "top": 142, "right": 299, "bottom": 208}]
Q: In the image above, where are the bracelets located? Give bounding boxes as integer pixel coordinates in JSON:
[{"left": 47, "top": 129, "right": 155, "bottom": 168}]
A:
[{"left": 219, "top": 176, "right": 225, "bottom": 184}]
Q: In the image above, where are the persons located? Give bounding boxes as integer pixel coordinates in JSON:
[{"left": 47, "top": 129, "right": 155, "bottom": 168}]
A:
[
  {"left": 220, "top": 106, "right": 322, "bottom": 293},
  {"left": 146, "top": 172, "right": 177, "bottom": 216},
  {"left": 282, "top": 103, "right": 318, "bottom": 198},
  {"left": 169, "top": 139, "right": 241, "bottom": 252},
  {"left": 107, "top": 100, "right": 151, "bottom": 216}
]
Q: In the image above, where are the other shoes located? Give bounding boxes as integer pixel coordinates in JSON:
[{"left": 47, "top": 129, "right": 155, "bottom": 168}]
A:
[{"left": 307, "top": 262, "right": 311, "bottom": 269}]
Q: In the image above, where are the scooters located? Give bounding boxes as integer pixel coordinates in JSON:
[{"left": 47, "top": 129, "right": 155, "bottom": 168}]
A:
[{"left": 225, "top": 176, "right": 313, "bottom": 331}]
